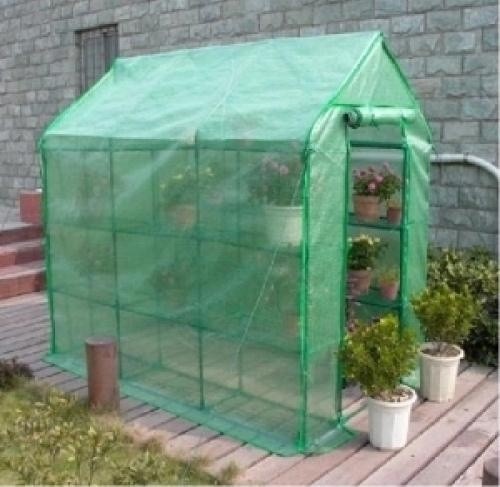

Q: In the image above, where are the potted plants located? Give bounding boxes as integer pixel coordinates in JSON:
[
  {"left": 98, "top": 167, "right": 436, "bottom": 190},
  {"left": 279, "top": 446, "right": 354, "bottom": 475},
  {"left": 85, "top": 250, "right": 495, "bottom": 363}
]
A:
[
  {"left": 407, "top": 274, "right": 474, "bottom": 401},
  {"left": 387, "top": 196, "right": 405, "bottom": 224},
  {"left": 260, "top": 206, "right": 302, "bottom": 247},
  {"left": 379, "top": 270, "right": 399, "bottom": 301},
  {"left": 344, "top": 309, "right": 419, "bottom": 453}
]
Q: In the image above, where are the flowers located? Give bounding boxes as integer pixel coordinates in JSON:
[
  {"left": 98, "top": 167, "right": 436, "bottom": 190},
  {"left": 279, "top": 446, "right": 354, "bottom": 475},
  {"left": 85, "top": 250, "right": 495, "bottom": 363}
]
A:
[
  {"left": 161, "top": 162, "right": 214, "bottom": 201},
  {"left": 70, "top": 244, "right": 298, "bottom": 343},
  {"left": 347, "top": 233, "right": 390, "bottom": 272},
  {"left": 351, "top": 162, "right": 401, "bottom": 201},
  {"left": 239, "top": 154, "right": 305, "bottom": 208},
  {"left": 63, "top": 162, "right": 110, "bottom": 224}
]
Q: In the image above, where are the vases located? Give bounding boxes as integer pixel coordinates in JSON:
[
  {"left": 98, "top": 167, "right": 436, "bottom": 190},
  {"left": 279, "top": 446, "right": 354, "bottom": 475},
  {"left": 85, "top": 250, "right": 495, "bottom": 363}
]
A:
[
  {"left": 168, "top": 205, "right": 196, "bottom": 231},
  {"left": 82, "top": 195, "right": 107, "bottom": 223},
  {"left": 352, "top": 193, "right": 384, "bottom": 223},
  {"left": 347, "top": 268, "right": 371, "bottom": 297}
]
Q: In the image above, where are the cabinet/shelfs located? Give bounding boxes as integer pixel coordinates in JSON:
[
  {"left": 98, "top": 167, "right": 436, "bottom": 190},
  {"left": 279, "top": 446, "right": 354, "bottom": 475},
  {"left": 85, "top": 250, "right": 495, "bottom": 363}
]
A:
[
  {"left": 46, "top": 137, "right": 340, "bottom": 449},
  {"left": 334, "top": 209, "right": 405, "bottom": 310}
]
